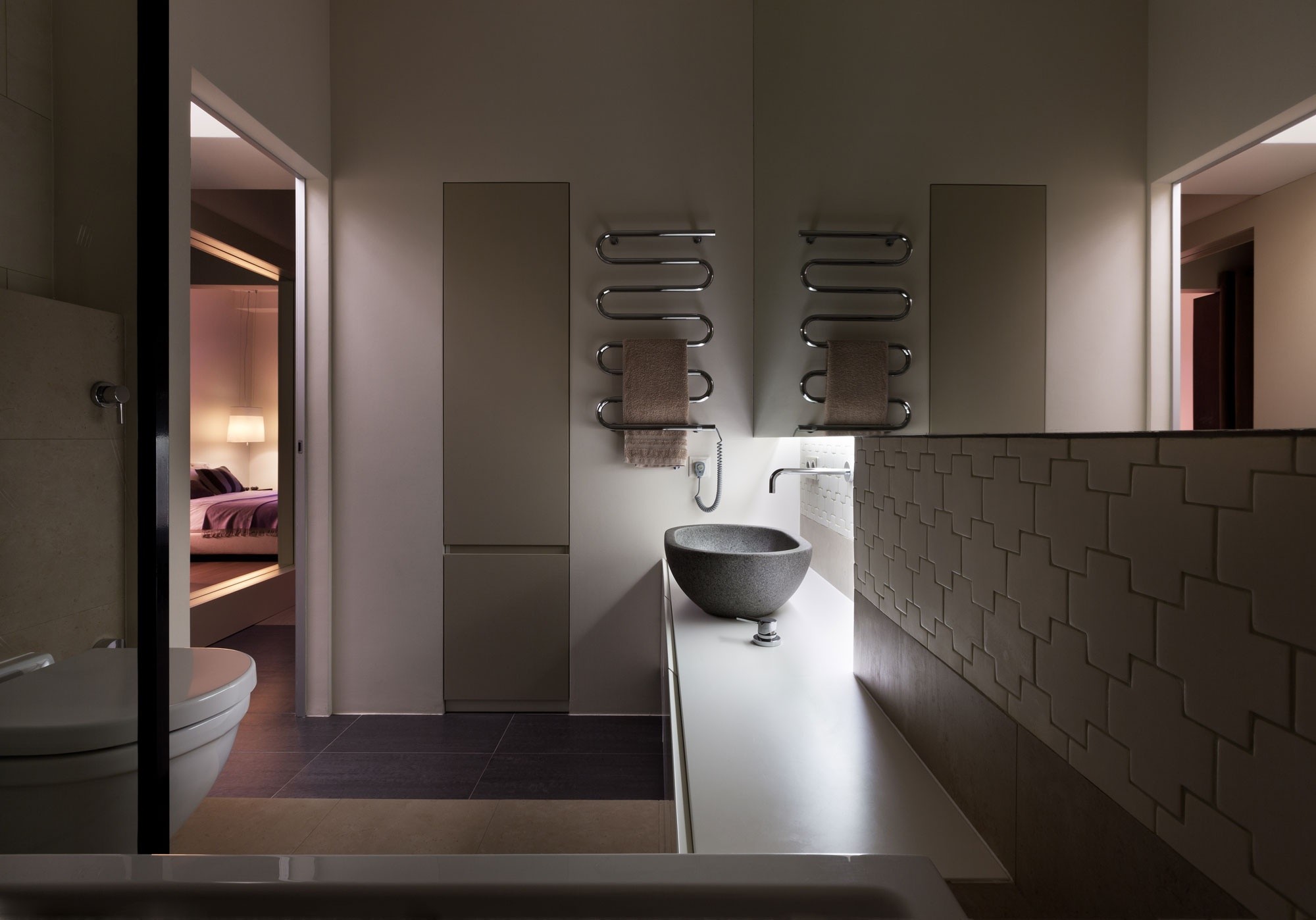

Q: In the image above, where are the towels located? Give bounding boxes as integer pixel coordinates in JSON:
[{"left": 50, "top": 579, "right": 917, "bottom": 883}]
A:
[
  {"left": 825, "top": 340, "right": 888, "bottom": 436},
  {"left": 622, "top": 339, "right": 689, "bottom": 469}
]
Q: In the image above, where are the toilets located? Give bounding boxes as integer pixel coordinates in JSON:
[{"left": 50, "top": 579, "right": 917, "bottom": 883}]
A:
[{"left": 0, "top": 641, "right": 257, "bottom": 854}]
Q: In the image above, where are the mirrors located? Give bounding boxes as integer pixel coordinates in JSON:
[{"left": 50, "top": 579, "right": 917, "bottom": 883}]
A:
[{"left": 750, "top": 0, "right": 1316, "bottom": 438}]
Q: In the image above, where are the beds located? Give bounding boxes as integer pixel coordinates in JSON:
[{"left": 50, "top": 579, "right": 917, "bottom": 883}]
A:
[{"left": 190, "top": 462, "right": 278, "bottom": 555}]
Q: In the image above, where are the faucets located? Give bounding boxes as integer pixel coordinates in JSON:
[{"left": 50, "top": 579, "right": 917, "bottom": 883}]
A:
[{"left": 769, "top": 462, "right": 854, "bottom": 493}]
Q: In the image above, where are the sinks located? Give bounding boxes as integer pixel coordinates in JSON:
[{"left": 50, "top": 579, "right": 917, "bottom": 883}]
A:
[{"left": 665, "top": 524, "right": 812, "bottom": 618}]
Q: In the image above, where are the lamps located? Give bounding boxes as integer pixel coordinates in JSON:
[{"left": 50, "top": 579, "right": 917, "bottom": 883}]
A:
[{"left": 227, "top": 407, "right": 265, "bottom": 446}]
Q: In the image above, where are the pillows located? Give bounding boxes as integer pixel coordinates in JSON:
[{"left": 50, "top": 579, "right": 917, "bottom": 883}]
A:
[
  {"left": 190, "top": 480, "right": 215, "bottom": 499},
  {"left": 194, "top": 466, "right": 246, "bottom": 496}
]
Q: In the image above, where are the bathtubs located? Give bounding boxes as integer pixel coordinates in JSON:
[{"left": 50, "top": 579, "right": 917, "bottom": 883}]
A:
[{"left": 0, "top": 854, "right": 969, "bottom": 920}]
